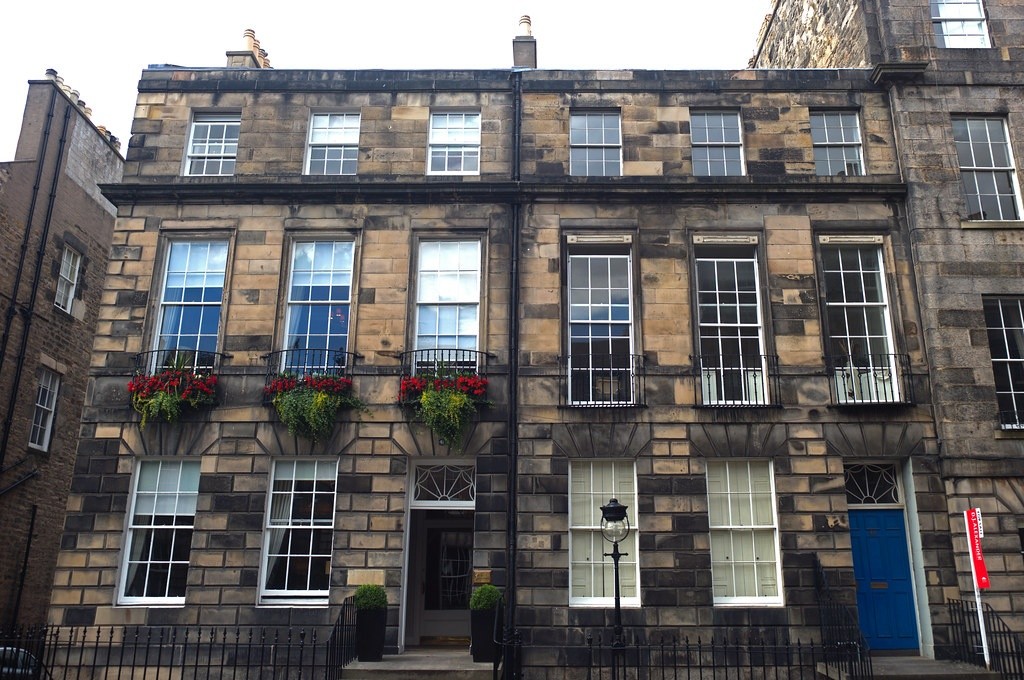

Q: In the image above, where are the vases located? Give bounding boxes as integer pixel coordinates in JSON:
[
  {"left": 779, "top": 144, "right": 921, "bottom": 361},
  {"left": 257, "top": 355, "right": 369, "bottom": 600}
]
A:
[
  {"left": 398, "top": 379, "right": 488, "bottom": 404},
  {"left": 127, "top": 383, "right": 219, "bottom": 407},
  {"left": 262, "top": 378, "right": 350, "bottom": 406}
]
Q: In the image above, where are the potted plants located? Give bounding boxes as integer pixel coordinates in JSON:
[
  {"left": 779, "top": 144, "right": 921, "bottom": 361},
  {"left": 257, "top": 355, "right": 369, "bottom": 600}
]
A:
[
  {"left": 469, "top": 584, "right": 504, "bottom": 663},
  {"left": 353, "top": 583, "right": 388, "bottom": 663}
]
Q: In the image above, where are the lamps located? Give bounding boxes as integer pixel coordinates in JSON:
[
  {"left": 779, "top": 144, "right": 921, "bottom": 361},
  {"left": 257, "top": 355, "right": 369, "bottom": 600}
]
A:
[
  {"left": 599, "top": 498, "right": 630, "bottom": 544},
  {"left": 330, "top": 308, "right": 344, "bottom": 324}
]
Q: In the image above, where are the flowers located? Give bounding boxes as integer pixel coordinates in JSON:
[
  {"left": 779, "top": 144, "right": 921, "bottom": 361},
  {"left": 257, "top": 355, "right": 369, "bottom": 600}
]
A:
[
  {"left": 127, "top": 352, "right": 218, "bottom": 432},
  {"left": 397, "top": 356, "right": 496, "bottom": 456},
  {"left": 263, "top": 370, "right": 374, "bottom": 442}
]
